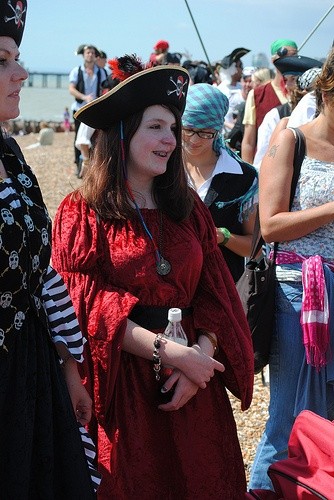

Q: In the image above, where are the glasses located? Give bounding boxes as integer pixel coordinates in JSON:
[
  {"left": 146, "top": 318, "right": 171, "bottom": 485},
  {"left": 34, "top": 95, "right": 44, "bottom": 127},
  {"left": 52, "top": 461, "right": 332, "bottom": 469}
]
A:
[{"left": 182, "top": 129, "right": 217, "bottom": 139}]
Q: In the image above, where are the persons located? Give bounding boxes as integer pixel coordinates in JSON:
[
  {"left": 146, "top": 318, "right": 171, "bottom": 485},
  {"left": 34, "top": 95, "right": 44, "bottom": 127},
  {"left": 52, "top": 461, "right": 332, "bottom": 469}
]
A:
[
  {"left": 252, "top": 55, "right": 323, "bottom": 173},
  {"left": 239, "top": 38, "right": 298, "bottom": 165},
  {"left": 0, "top": 0, "right": 102, "bottom": 500},
  {"left": 249, "top": 41, "right": 334, "bottom": 500},
  {"left": 286, "top": 40, "right": 334, "bottom": 128},
  {"left": 269, "top": 68, "right": 322, "bottom": 147},
  {"left": 184, "top": 84, "right": 259, "bottom": 287},
  {"left": 69, "top": 41, "right": 276, "bottom": 180},
  {"left": 52, "top": 70, "right": 254, "bottom": 500}
]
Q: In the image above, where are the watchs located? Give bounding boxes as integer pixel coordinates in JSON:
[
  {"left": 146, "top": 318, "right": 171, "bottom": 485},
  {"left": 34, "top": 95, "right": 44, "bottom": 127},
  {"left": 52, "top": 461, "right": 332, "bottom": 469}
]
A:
[{"left": 218, "top": 228, "right": 230, "bottom": 245}]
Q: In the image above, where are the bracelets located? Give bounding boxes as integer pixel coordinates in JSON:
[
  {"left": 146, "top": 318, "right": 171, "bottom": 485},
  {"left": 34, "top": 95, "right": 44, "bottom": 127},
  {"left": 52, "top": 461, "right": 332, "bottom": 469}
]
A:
[
  {"left": 152, "top": 332, "right": 164, "bottom": 379},
  {"left": 200, "top": 332, "right": 217, "bottom": 353}
]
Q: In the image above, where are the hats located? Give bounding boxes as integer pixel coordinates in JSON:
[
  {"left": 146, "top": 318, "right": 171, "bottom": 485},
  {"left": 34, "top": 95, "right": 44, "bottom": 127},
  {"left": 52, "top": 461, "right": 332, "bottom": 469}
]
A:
[
  {"left": 273, "top": 55, "right": 323, "bottom": 76},
  {"left": 73, "top": 66, "right": 190, "bottom": 131},
  {"left": 271, "top": 39, "right": 296, "bottom": 55},
  {"left": 154, "top": 40, "right": 170, "bottom": 51},
  {"left": 97, "top": 52, "right": 106, "bottom": 58},
  {"left": 0, "top": 0, "right": 27, "bottom": 47},
  {"left": 297, "top": 67, "right": 323, "bottom": 88}
]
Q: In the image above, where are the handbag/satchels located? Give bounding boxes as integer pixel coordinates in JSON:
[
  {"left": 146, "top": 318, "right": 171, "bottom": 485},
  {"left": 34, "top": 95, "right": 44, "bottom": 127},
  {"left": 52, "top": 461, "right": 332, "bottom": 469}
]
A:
[{"left": 235, "top": 259, "right": 277, "bottom": 374}]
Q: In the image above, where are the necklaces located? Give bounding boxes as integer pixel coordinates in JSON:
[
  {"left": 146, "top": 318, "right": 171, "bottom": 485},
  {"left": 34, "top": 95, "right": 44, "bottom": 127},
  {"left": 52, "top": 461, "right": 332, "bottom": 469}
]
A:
[{"left": 126, "top": 182, "right": 171, "bottom": 276}]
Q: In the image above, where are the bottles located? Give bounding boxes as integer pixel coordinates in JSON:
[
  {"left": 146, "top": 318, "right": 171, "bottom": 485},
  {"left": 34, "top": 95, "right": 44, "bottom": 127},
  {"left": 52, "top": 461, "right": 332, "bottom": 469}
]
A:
[{"left": 159, "top": 308, "right": 188, "bottom": 384}]
